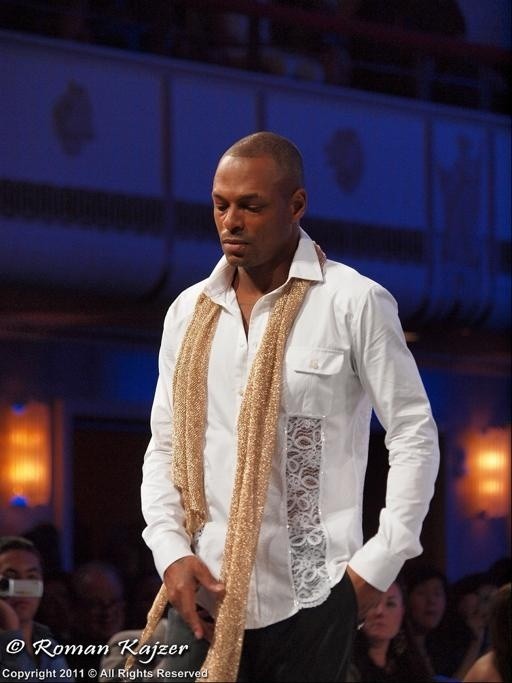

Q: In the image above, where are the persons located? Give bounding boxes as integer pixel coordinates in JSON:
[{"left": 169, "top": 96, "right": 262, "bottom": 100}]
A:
[
  {"left": 1, "top": 533, "right": 512, "bottom": 682},
  {"left": 139, "top": 131, "right": 442, "bottom": 683}
]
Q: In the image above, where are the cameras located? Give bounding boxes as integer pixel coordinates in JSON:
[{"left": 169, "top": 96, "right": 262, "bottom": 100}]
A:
[{"left": 0, "top": 575, "right": 43, "bottom": 597}]
